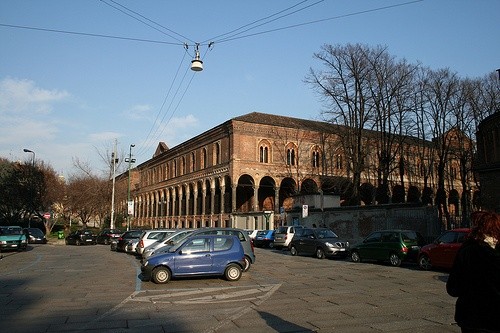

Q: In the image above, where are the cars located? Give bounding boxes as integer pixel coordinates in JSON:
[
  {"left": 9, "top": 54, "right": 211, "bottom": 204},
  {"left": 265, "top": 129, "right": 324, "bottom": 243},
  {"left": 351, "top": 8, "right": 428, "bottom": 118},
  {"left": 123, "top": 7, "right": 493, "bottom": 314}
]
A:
[
  {"left": 347, "top": 230, "right": 426, "bottom": 267},
  {"left": 0, "top": 226, "right": 27, "bottom": 251},
  {"left": 140, "top": 235, "right": 245, "bottom": 284},
  {"left": 418, "top": 228, "right": 473, "bottom": 271},
  {"left": 254, "top": 230, "right": 277, "bottom": 249},
  {"left": 288, "top": 228, "right": 350, "bottom": 259},
  {"left": 95, "top": 229, "right": 124, "bottom": 245},
  {"left": 243, "top": 229, "right": 263, "bottom": 247}
]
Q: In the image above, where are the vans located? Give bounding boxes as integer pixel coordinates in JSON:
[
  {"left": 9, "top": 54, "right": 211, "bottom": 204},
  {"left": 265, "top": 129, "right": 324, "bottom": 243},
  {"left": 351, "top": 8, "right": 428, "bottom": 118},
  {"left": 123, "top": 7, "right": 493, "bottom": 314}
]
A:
[
  {"left": 24, "top": 228, "right": 46, "bottom": 244},
  {"left": 111, "top": 228, "right": 256, "bottom": 272},
  {"left": 274, "top": 225, "right": 312, "bottom": 250}
]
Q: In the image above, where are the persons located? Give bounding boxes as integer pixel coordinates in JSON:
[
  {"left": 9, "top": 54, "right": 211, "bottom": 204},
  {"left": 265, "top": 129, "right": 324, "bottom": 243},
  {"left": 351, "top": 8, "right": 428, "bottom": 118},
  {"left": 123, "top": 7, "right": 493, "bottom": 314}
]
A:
[{"left": 446, "top": 211, "right": 500, "bottom": 333}]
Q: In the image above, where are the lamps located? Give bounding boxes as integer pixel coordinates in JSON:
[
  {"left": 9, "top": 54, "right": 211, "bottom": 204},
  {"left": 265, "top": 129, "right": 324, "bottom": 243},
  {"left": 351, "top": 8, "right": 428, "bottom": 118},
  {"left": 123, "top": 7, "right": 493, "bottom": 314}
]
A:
[{"left": 191, "top": 43, "right": 203, "bottom": 71}]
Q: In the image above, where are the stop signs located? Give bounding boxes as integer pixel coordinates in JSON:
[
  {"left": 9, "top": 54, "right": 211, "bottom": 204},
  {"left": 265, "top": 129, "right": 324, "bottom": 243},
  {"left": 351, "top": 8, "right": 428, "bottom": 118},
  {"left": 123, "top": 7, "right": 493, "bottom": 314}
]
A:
[{"left": 44, "top": 213, "right": 51, "bottom": 220}]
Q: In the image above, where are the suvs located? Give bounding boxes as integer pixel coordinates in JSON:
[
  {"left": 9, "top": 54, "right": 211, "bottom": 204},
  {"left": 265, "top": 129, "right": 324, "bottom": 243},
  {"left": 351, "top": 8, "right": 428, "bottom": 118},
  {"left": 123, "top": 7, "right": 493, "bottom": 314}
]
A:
[{"left": 65, "top": 230, "right": 97, "bottom": 246}]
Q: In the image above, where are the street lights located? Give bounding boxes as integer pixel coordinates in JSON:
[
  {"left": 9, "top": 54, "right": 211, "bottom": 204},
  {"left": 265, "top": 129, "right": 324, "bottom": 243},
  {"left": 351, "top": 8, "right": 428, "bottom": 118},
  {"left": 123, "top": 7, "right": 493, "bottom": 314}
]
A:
[
  {"left": 23, "top": 149, "right": 35, "bottom": 227},
  {"left": 263, "top": 210, "right": 271, "bottom": 230},
  {"left": 127, "top": 144, "right": 135, "bottom": 231}
]
